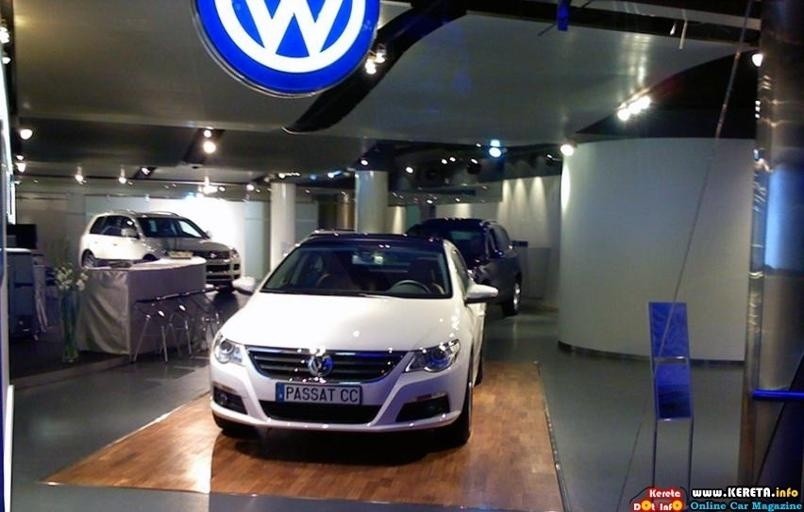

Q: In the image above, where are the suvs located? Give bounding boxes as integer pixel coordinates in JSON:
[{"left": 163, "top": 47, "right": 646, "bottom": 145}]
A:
[
  {"left": 77, "top": 210, "right": 241, "bottom": 292},
  {"left": 398, "top": 219, "right": 522, "bottom": 314}
]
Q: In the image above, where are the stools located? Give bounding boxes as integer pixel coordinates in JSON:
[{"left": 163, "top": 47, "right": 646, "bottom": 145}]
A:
[{"left": 132, "top": 285, "right": 227, "bottom": 369}]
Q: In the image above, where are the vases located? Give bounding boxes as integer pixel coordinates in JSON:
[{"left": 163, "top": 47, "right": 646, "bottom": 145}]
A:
[{"left": 55, "top": 287, "right": 84, "bottom": 364}]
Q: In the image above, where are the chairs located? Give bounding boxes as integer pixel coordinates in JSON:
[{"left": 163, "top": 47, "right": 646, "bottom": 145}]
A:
[
  {"left": 405, "top": 259, "right": 444, "bottom": 296},
  {"left": 306, "top": 249, "right": 375, "bottom": 295}
]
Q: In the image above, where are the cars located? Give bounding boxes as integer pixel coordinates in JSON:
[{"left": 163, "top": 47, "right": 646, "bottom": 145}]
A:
[{"left": 206, "top": 227, "right": 499, "bottom": 448}]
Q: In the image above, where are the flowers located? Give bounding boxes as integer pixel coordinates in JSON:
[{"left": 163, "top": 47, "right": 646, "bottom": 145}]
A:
[{"left": 41, "top": 260, "right": 94, "bottom": 367}]
